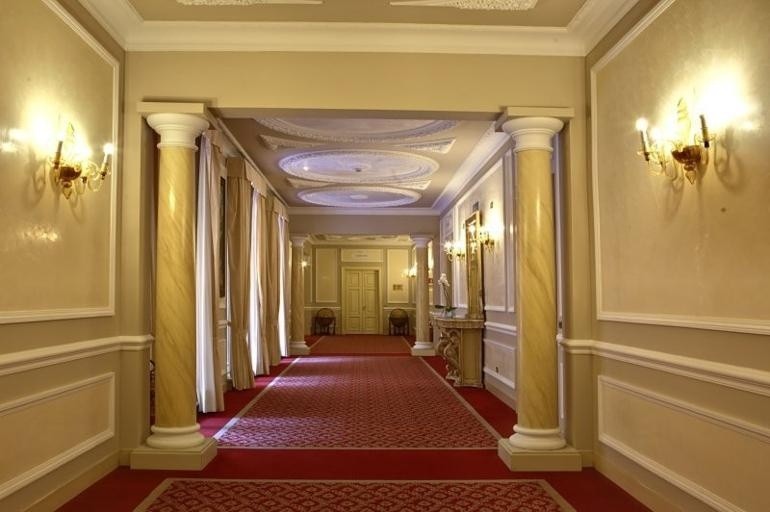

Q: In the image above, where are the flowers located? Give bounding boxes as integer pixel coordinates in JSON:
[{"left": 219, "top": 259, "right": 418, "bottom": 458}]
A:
[{"left": 435, "top": 271, "right": 458, "bottom": 312}]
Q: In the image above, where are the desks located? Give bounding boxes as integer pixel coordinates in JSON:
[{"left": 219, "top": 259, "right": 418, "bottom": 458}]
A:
[{"left": 435, "top": 318, "right": 484, "bottom": 389}]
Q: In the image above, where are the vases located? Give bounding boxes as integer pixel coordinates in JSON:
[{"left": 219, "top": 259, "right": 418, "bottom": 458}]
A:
[{"left": 439, "top": 311, "right": 452, "bottom": 319}]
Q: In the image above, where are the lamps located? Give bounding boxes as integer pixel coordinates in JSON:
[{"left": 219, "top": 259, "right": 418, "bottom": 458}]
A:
[
  {"left": 441, "top": 225, "right": 497, "bottom": 264},
  {"left": 48, "top": 120, "right": 115, "bottom": 199},
  {"left": 635, "top": 96, "right": 717, "bottom": 184}
]
